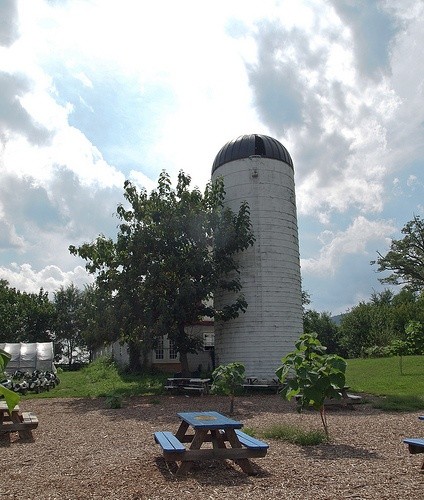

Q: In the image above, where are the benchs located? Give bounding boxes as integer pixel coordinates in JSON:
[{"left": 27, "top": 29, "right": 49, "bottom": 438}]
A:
[
  {"left": 163, "top": 374, "right": 362, "bottom": 412},
  {"left": 153, "top": 431, "right": 188, "bottom": 477},
  {"left": 403, "top": 437, "right": 424, "bottom": 470},
  {"left": 21, "top": 410, "right": 40, "bottom": 442},
  {"left": 220, "top": 428, "right": 269, "bottom": 476}
]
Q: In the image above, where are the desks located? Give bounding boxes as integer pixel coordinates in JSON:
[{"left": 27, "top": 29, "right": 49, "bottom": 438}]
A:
[
  {"left": 166, "top": 410, "right": 258, "bottom": 478},
  {"left": 0, "top": 400, "right": 34, "bottom": 441}
]
